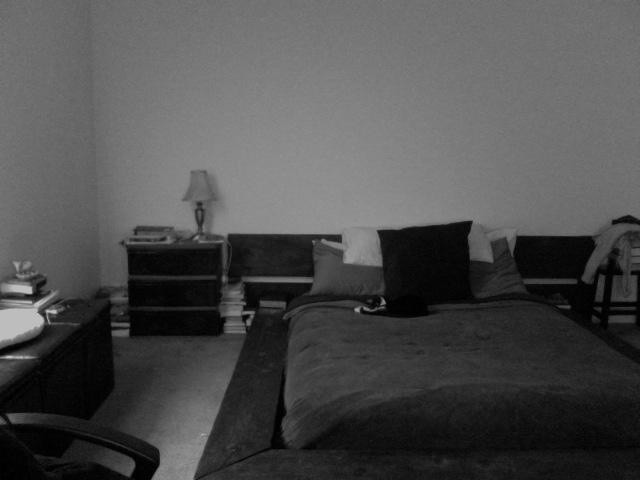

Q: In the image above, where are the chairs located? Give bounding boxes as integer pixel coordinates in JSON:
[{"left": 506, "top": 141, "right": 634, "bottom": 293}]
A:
[{"left": 0, "top": 411, "right": 160, "bottom": 480}]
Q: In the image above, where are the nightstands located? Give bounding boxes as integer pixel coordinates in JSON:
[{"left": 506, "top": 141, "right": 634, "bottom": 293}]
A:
[{"left": 126, "top": 235, "right": 225, "bottom": 338}]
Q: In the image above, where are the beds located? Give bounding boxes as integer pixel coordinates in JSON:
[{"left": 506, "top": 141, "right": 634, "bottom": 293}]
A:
[{"left": 195, "top": 232, "right": 640, "bottom": 480}]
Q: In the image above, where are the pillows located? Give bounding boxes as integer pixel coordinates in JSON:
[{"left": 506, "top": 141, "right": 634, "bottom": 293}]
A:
[{"left": 302, "top": 220, "right": 530, "bottom": 318}]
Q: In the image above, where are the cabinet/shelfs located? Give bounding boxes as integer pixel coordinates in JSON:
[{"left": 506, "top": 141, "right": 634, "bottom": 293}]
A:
[{"left": 0, "top": 296, "right": 117, "bottom": 459}]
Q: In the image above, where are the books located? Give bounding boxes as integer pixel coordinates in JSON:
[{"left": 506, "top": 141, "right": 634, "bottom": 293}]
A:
[
  {"left": 96, "top": 284, "right": 131, "bottom": 339},
  {"left": 1, "top": 272, "right": 91, "bottom": 326},
  {"left": 624, "top": 233, "right": 640, "bottom": 273},
  {"left": 126, "top": 224, "right": 177, "bottom": 246},
  {"left": 219, "top": 281, "right": 256, "bottom": 336}
]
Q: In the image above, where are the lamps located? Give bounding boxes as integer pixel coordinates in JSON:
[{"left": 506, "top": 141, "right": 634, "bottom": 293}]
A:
[{"left": 182, "top": 169, "right": 217, "bottom": 241}]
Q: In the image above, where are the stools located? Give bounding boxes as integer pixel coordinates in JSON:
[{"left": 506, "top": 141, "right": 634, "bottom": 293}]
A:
[{"left": 584, "top": 264, "right": 639, "bottom": 331}]
